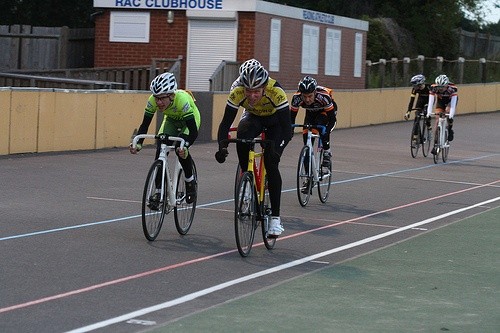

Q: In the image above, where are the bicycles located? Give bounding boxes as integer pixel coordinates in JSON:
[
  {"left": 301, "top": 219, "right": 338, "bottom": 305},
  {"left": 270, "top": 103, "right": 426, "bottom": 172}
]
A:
[
  {"left": 430, "top": 111, "right": 450, "bottom": 163},
  {"left": 226, "top": 135, "right": 279, "bottom": 259},
  {"left": 291, "top": 123, "right": 335, "bottom": 206},
  {"left": 405, "top": 108, "right": 432, "bottom": 159},
  {"left": 130, "top": 133, "right": 198, "bottom": 240}
]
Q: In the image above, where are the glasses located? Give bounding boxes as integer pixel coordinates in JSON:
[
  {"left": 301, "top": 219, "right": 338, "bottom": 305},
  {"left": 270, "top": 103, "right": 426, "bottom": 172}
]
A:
[{"left": 154, "top": 94, "right": 171, "bottom": 101}]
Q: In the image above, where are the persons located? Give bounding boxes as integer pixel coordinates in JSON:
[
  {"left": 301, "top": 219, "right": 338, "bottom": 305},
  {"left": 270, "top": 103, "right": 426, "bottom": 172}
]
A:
[
  {"left": 289, "top": 76, "right": 337, "bottom": 195},
  {"left": 215, "top": 58, "right": 292, "bottom": 237},
  {"left": 130, "top": 72, "right": 201, "bottom": 211},
  {"left": 426, "top": 75, "right": 458, "bottom": 154},
  {"left": 404, "top": 74, "right": 432, "bottom": 147}
]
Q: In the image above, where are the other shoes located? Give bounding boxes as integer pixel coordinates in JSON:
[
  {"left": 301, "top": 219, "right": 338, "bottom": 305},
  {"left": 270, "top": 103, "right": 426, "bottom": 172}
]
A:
[
  {"left": 185, "top": 179, "right": 197, "bottom": 204},
  {"left": 322, "top": 152, "right": 332, "bottom": 167},
  {"left": 148, "top": 192, "right": 161, "bottom": 201},
  {"left": 447, "top": 129, "right": 454, "bottom": 141},
  {"left": 431, "top": 148, "right": 436, "bottom": 156},
  {"left": 410, "top": 143, "right": 419, "bottom": 149},
  {"left": 301, "top": 182, "right": 309, "bottom": 192}
]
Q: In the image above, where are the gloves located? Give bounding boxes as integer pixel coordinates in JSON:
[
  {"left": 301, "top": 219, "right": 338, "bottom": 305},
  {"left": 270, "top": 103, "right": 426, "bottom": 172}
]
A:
[
  {"left": 215, "top": 149, "right": 229, "bottom": 164},
  {"left": 320, "top": 126, "right": 331, "bottom": 140},
  {"left": 271, "top": 151, "right": 281, "bottom": 163},
  {"left": 403, "top": 113, "right": 410, "bottom": 121}
]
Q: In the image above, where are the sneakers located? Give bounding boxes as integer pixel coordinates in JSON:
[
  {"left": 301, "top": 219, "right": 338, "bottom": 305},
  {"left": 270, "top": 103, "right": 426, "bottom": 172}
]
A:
[{"left": 267, "top": 217, "right": 284, "bottom": 235}]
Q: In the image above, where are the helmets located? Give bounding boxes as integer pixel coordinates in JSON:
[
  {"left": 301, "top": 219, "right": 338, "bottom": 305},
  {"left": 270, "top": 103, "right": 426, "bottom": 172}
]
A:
[
  {"left": 298, "top": 75, "right": 317, "bottom": 93},
  {"left": 410, "top": 75, "right": 425, "bottom": 85},
  {"left": 149, "top": 72, "right": 177, "bottom": 95},
  {"left": 238, "top": 59, "right": 268, "bottom": 88},
  {"left": 435, "top": 75, "right": 449, "bottom": 87}
]
